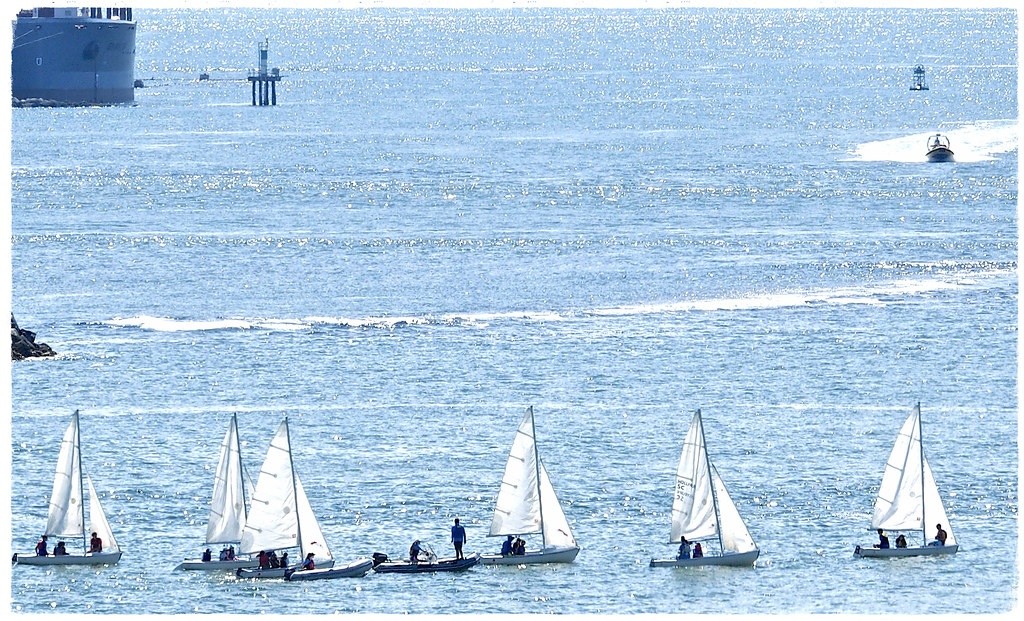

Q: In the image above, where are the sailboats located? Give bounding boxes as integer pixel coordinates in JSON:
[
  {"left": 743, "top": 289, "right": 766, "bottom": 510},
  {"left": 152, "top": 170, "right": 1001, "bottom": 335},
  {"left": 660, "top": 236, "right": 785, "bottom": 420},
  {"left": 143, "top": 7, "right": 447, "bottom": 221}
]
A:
[
  {"left": 14, "top": 409, "right": 124, "bottom": 565},
  {"left": 173, "top": 412, "right": 291, "bottom": 573},
  {"left": 647, "top": 408, "right": 761, "bottom": 570},
  {"left": 477, "top": 405, "right": 581, "bottom": 565},
  {"left": 850, "top": 401, "right": 959, "bottom": 560},
  {"left": 237, "top": 410, "right": 338, "bottom": 577}
]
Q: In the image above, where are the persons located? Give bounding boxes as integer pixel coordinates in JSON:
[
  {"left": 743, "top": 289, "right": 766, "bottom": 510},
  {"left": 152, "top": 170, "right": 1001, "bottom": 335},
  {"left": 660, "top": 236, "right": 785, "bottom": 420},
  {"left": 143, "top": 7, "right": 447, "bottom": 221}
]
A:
[
  {"left": 302, "top": 553, "right": 315, "bottom": 570},
  {"left": 35, "top": 535, "right": 50, "bottom": 556},
  {"left": 678, "top": 536, "right": 690, "bottom": 559},
  {"left": 513, "top": 538, "right": 526, "bottom": 555},
  {"left": 693, "top": 543, "right": 703, "bottom": 558},
  {"left": 896, "top": 535, "right": 906, "bottom": 548},
  {"left": 935, "top": 137, "right": 939, "bottom": 144},
  {"left": 935, "top": 524, "right": 946, "bottom": 546},
  {"left": 87, "top": 532, "right": 102, "bottom": 553},
  {"left": 451, "top": 518, "right": 466, "bottom": 559},
  {"left": 221, "top": 546, "right": 234, "bottom": 561},
  {"left": 410, "top": 540, "right": 426, "bottom": 561},
  {"left": 260, "top": 551, "right": 270, "bottom": 568},
  {"left": 281, "top": 553, "right": 289, "bottom": 567},
  {"left": 501, "top": 536, "right": 514, "bottom": 556},
  {"left": 203, "top": 549, "right": 212, "bottom": 562},
  {"left": 53, "top": 542, "right": 69, "bottom": 555},
  {"left": 270, "top": 554, "right": 279, "bottom": 568},
  {"left": 874, "top": 529, "right": 889, "bottom": 549}
]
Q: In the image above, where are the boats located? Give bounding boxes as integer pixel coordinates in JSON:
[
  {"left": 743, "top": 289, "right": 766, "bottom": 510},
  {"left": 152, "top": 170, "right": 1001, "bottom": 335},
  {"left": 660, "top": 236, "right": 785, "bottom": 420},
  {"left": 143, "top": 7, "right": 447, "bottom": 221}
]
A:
[
  {"left": 925, "top": 133, "right": 955, "bottom": 163},
  {"left": 283, "top": 557, "right": 374, "bottom": 582},
  {"left": 370, "top": 540, "right": 484, "bottom": 574}
]
